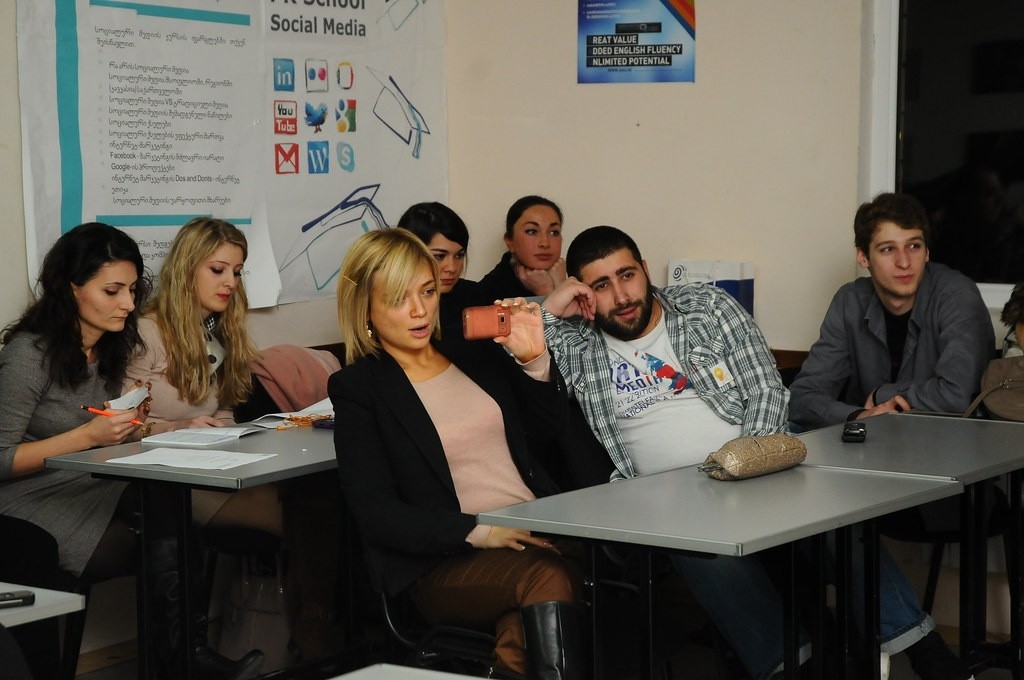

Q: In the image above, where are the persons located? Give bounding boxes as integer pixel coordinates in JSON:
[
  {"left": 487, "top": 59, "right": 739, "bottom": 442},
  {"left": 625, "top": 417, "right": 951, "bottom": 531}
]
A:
[
  {"left": 786, "top": 191, "right": 1002, "bottom": 493},
  {"left": 0, "top": 196, "right": 978, "bottom": 680},
  {"left": 999, "top": 281, "right": 1024, "bottom": 358}
]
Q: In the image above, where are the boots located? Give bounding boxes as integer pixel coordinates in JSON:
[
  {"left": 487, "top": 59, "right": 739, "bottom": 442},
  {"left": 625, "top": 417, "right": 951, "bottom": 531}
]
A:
[
  {"left": 280, "top": 497, "right": 345, "bottom": 671},
  {"left": 142, "top": 535, "right": 263, "bottom": 680},
  {"left": 521, "top": 602, "right": 584, "bottom": 680}
]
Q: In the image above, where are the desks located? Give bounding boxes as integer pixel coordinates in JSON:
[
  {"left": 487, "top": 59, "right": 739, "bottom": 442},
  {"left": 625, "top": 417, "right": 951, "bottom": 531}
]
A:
[
  {"left": 475, "top": 464, "right": 965, "bottom": 680},
  {"left": 795, "top": 413, "right": 1024, "bottom": 680},
  {"left": 44, "top": 423, "right": 340, "bottom": 680}
]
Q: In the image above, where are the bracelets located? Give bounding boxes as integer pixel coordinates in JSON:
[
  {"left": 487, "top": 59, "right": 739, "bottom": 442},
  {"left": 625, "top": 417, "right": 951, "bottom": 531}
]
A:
[{"left": 143, "top": 421, "right": 155, "bottom": 438}]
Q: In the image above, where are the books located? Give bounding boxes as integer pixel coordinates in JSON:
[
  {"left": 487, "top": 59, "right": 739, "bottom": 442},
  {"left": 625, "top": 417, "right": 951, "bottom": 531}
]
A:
[{"left": 141, "top": 427, "right": 261, "bottom": 446}]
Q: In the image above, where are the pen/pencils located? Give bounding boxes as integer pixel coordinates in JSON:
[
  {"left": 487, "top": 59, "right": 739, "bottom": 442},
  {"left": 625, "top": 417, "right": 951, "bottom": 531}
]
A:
[{"left": 79, "top": 404, "right": 146, "bottom": 427}]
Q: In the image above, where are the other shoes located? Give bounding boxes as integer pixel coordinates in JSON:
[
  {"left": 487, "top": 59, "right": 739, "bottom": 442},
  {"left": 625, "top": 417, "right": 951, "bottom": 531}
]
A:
[
  {"left": 907, "top": 630, "right": 975, "bottom": 680},
  {"left": 767, "top": 649, "right": 871, "bottom": 680}
]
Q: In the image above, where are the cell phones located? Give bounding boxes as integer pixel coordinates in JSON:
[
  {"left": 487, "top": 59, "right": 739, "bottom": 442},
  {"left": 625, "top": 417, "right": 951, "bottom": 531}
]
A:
[
  {"left": 462, "top": 305, "right": 513, "bottom": 340},
  {"left": 841, "top": 421, "right": 866, "bottom": 443}
]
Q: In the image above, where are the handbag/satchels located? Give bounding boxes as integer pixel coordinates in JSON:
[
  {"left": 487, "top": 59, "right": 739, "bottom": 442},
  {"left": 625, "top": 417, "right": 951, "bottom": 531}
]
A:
[
  {"left": 696, "top": 434, "right": 807, "bottom": 481},
  {"left": 962, "top": 354, "right": 1024, "bottom": 421}
]
